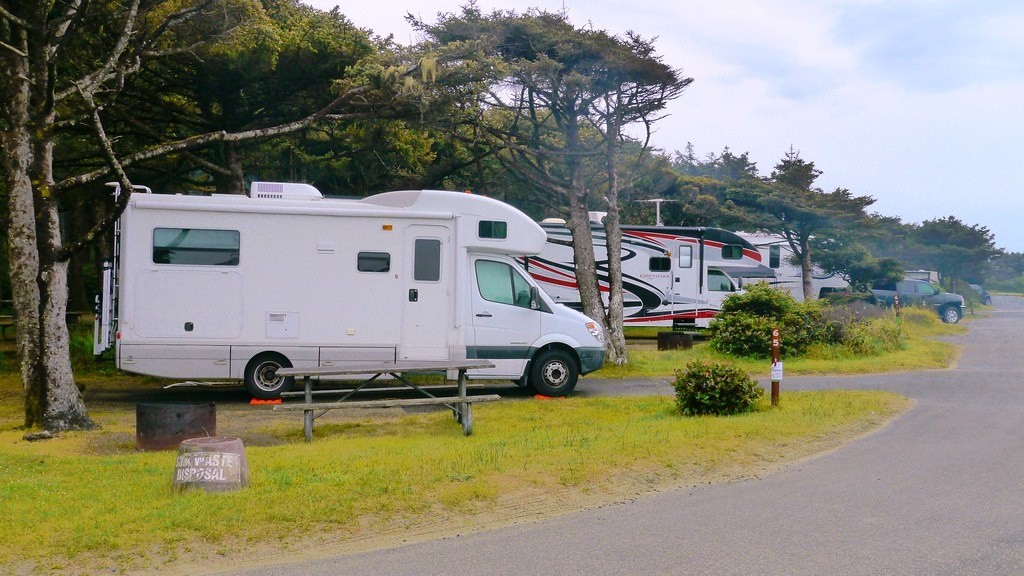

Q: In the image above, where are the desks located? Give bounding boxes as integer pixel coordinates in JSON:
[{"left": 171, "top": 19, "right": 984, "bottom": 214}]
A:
[{"left": 272, "top": 359, "right": 495, "bottom": 437}]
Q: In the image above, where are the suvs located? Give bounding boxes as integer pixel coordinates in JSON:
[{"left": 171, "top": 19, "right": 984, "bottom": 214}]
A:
[{"left": 970, "top": 283, "right": 991, "bottom": 306}]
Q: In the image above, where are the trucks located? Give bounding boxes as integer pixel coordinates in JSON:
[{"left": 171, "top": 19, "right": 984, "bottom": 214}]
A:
[
  {"left": 513, "top": 211, "right": 776, "bottom": 329},
  {"left": 93, "top": 182, "right": 608, "bottom": 397}
]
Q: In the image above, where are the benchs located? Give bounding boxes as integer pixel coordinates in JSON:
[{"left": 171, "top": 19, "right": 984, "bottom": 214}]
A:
[{"left": 273, "top": 384, "right": 505, "bottom": 443}]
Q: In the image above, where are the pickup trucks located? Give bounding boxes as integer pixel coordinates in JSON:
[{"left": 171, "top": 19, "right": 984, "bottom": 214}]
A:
[{"left": 863, "top": 280, "right": 965, "bottom": 323}]
